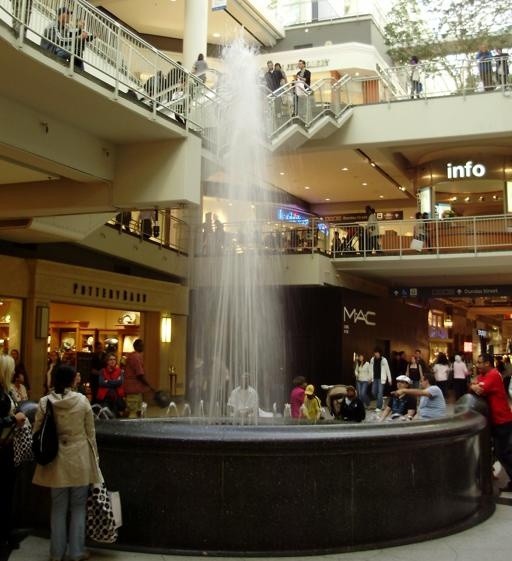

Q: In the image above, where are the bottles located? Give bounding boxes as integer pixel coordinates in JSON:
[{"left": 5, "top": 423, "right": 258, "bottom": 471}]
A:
[
  {"left": 304, "top": 385, "right": 314, "bottom": 396},
  {"left": 396, "top": 374, "right": 412, "bottom": 385}
]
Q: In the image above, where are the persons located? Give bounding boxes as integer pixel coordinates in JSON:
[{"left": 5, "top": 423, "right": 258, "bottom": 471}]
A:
[
  {"left": 192, "top": 351, "right": 258, "bottom": 417},
  {"left": 115, "top": 206, "right": 451, "bottom": 257},
  {"left": 40, "top": 6, "right": 93, "bottom": 71},
  {"left": 409, "top": 56, "right": 421, "bottom": 99},
  {"left": 43, "top": 342, "right": 125, "bottom": 415},
  {"left": 33, "top": 365, "right": 104, "bottom": 561},
  {"left": 0, "top": 350, "right": 29, "bottom": 545},
  {"left": 469, "top": 353, "right": 512, "bottom": 491},
  {"left": 494, "top": 48, "right": 509, "bottom": 88},
  {"left": 262, "top": 59, "right": 311, "bottom": 117},
  {"left": 125, "top": 54, "right": 208, "bottom": 125},
  {"left": 123, "top": 339, "right": 154, "bottom": 417},
  {"left": 290, "top": 373, "right": 447, "bottom": 421},
  {"left": 476, "top": 45, "right": 494, "bottom": 90},
  {"left": 353, "top": 349, "right": 510, "bottom": 402}
]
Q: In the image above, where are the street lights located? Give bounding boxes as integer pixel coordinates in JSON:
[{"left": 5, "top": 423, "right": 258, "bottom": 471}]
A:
[
  {"left": 13, "top": 416, "right": 35, "bottom": 466},
  {"left": 33, "top": 415, "right": 57, "bottom": 464},
  {"left": 87, "top": 483, "right": 124, "bottom": 543}
]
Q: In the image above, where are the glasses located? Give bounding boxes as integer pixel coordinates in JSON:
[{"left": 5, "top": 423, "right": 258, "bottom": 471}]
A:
[
  {"left": 161, "top": 316, "right": 173, "bottom": 343},
  {"left": 35, "top": 306, "right": 50, "bottom": 338}
]
